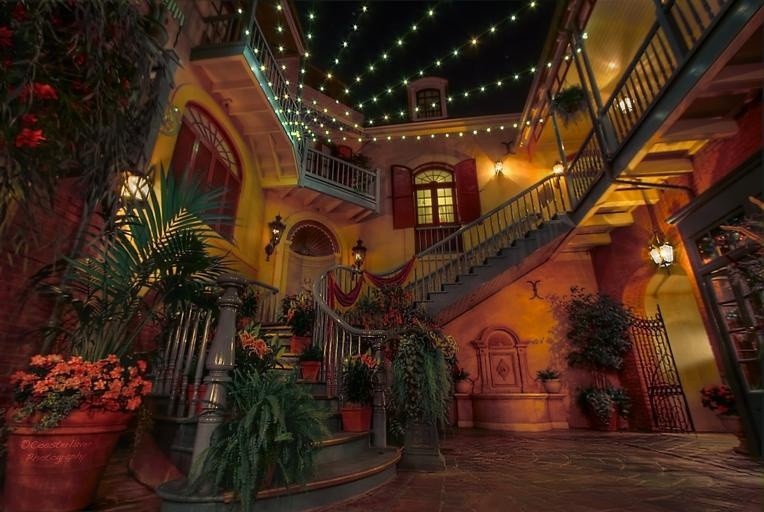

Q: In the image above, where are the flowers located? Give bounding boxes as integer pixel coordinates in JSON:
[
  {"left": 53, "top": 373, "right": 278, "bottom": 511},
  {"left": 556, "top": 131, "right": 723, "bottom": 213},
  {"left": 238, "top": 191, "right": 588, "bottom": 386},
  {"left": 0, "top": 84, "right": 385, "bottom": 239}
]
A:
[
  {"left": 700, "top": 384, "right": 738, "bottom": 416},
  {"left": 9, "top": 354, "right": 152, "bottom": 432}
]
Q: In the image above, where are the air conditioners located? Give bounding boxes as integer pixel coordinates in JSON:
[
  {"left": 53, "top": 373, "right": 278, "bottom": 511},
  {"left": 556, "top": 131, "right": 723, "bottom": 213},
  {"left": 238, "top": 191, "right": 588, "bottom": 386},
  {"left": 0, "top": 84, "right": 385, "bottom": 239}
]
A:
[{"left": 287, "top": 306, "right": 316, "bottom": 353}]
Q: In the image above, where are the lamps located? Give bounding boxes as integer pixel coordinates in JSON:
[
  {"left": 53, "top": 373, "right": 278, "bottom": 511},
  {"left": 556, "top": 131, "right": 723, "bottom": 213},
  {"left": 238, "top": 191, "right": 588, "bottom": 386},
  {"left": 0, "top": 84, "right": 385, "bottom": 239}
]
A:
[
  {"left": 642, "top": 188, "right": 673, "bottom": 275},
  {"left": 616, "top": 96, "right": 635, "bottom": 114},
  {"left": 120, "top": 170, "right": 152, "bottom": 209},
  {"left": 351, "top": 237, "right": 367, "bottom": 281},
  {"left": 265, "top": 212, "right": 286, "bottom": 261},
  {"left": 553, "top": 160, "right": 564, "bottom": 189},
  {"left": 495, "top": 160, "right": 504, "bottom": 175}
]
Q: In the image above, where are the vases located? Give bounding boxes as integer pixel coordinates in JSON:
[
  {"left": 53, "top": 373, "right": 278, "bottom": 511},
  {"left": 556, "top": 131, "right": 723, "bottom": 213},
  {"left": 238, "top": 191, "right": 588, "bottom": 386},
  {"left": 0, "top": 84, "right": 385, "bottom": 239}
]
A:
[
  {"left": 718, "top": 414, "right": 752, "bottom": 455},
  {"left": 4, "top": 407, "right": 136, "bottom": 512}
]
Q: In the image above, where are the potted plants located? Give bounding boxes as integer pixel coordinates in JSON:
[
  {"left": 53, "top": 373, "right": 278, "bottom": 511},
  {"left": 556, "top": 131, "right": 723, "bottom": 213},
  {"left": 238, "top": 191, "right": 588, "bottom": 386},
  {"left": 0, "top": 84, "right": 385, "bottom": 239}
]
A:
[
  {"left": 298, "top": 344, "right": 324, "bottom": 382},
  {"left": 339, "top": 358, "right": 379, "bottom": 432},
  {"left": 560, "top": 286, "right": 636, "bottom": 432},
  {"left": 534, "top": 369, "right": 561, "bottom": 393},
  {"left": 452, "top": 368, "right": 471, "bottom": 393},
  {"left": 175, "top": 363, "right": 332, "bottom": 512}
]
